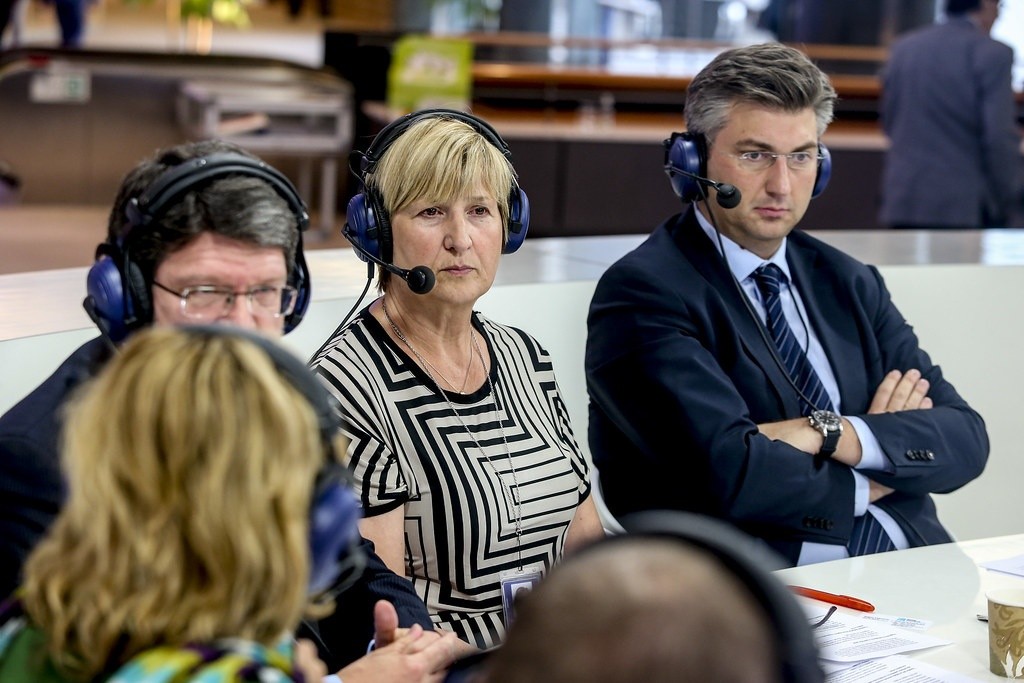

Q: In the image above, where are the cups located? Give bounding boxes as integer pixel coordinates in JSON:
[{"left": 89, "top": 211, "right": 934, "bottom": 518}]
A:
[{"left": 983, "top": 574, "right": 1023, "bottom": 679}]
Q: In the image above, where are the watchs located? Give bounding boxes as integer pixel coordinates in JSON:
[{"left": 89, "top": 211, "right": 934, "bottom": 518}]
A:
[{"left": 808, "top": 409, "right": 843, "bottom": 470}]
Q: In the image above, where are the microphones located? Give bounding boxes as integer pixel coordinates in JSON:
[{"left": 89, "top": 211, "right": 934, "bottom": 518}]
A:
[
  {"left": 664, "top": 165, "right": 741, "bottom": 208},
  {"left": 341, "top": 225, "right": 436, "bottom": 295}
]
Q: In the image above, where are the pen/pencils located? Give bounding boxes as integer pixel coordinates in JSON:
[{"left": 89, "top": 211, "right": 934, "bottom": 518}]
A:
[{"left": 787, "top": 585, "right": 875, "bottom": 613}]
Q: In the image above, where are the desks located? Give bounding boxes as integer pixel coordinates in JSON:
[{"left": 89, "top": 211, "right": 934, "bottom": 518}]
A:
[{"left": 780, "top": 532, "right": 1024, "bottom": 683}]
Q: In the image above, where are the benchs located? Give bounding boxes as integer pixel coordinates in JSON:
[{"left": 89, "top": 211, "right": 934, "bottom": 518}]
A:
[{"left": 374, "top": 24, "right": 888, "bottom": 226}]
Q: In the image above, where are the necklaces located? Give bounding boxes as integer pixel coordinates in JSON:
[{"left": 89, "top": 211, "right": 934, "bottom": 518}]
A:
[{"left": 382, "top": 295, "right": 524, "bottom": 574}]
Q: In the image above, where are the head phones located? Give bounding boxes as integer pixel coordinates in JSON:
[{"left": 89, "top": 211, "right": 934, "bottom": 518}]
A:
[
  {"left": 84, "top": 154, "right": 311, "bottom": 336},
  {"left": 663, "top": 131, "right": 832, "bottom": 200},
  {"left": 177, "top": 320, "right": 365, "bottom": 602},
  {"left": 346, "top": 107, "right": 530, "bottom": 270}
]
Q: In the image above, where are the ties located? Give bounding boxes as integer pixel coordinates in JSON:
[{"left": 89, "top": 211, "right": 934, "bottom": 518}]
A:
[{"left": 749, "top": 263, "right": 898, "bottom": 558}]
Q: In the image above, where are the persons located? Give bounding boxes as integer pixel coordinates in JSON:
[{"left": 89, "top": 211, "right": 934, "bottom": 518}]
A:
[
  {"left": 754, "top": 0, "right": 903, "bottom": 75},
  {"left": 584, "top": 42, "right": 990, "bottom": 572},
  {"left": 0, "top": 0, "right": 86, "bottom": 191},
  {"left": 0, "top": 136, "right": 457, "bottom": 683},
  {"left": 0, "top": 320, "right": 328, "bottom": 683},
  {"left": 304, "top": 102, "right": 608, "bottom": 683},
  {"left": 483, "top": 532, "right": 803, "bottom": 683},
  {"left": 879, "top": 0, "right": 1024, "bottom": 229}
]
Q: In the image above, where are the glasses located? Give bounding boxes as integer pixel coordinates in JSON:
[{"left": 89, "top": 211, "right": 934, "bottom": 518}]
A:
[
  {"left": 151, "top": 279, "right": 298, "bottom": 321},
  {"left": 705, "top": 134, "right": 827, "bottom": 171}
]
